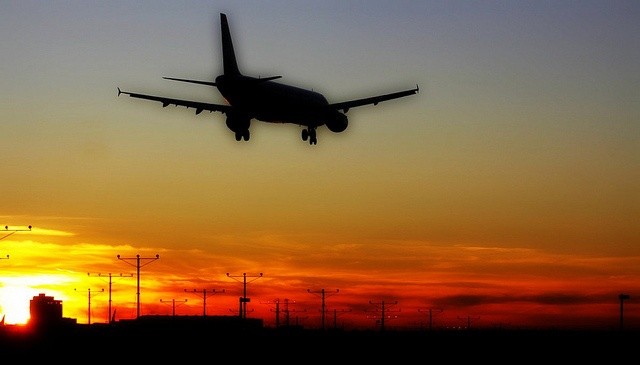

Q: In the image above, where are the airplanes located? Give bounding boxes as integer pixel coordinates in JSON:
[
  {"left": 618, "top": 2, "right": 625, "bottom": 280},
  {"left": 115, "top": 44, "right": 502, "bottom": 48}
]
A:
[{"left": 116, "top": 12, "right": 420, "bottom": 147}]
